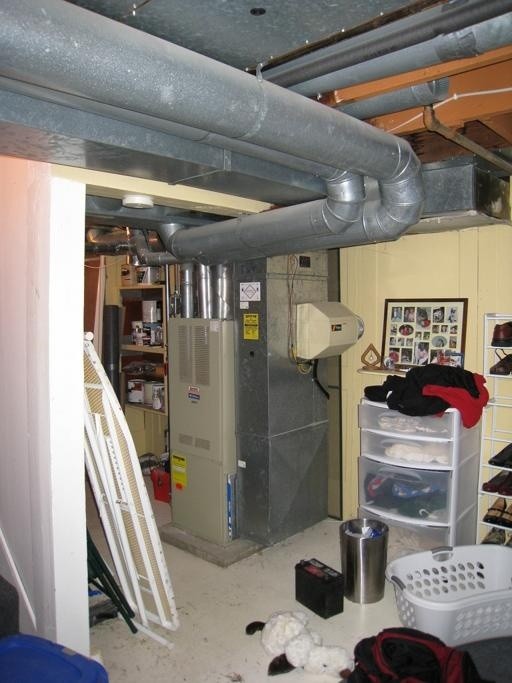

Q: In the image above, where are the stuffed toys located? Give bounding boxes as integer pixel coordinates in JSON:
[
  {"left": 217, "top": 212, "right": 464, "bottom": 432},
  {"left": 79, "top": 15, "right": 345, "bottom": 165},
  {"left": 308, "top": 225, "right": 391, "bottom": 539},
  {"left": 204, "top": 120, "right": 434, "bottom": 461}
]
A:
[{"left": 245, "top": 612, "right": 354, "bottom": 678}]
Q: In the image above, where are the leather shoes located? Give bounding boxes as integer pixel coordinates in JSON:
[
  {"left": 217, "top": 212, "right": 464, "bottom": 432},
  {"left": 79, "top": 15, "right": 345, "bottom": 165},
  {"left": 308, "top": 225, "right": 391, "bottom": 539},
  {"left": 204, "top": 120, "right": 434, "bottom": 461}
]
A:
[
  {"left": 482, "top": 497, "right": 507, "bottom": 524},
  {"left": 489, "top": 351, "right": 512, "bottom": 377},
  {"left": 482, "top": 470, "right": 510, "bottom": 493},
  {"left": 487, "top": 441, "right": 512, "bottom": 470},
  {"left": 498, "top": 503, "right": 512, "bottom": 528},
  {"left": 496, "top": 471, "right": 512, "bottom": 497},
  {"left": 491, "top": 321, "right": 512, "bottom": 347}
]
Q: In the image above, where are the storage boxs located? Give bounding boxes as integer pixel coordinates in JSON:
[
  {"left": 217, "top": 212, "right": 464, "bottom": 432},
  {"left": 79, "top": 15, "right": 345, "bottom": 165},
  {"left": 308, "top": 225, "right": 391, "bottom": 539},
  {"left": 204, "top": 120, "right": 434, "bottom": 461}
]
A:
[
  {"left": 0, "top": 630, "right": 109, "bottom": 683},
  {"left": 150, "top": 465, "right": 172, "bottom": 503}
]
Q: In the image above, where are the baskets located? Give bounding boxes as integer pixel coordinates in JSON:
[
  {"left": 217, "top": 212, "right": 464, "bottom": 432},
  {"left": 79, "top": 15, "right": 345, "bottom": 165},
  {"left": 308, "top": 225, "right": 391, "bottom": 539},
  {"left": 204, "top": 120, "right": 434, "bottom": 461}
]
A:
[{"left": 384, "top": 543, "right": 512, "bottom": 650}]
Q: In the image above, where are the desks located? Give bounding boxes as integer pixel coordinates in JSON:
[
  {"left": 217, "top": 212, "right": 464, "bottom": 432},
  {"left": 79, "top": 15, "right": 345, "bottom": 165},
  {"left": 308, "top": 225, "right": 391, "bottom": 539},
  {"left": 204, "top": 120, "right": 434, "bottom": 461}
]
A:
[{"left": 79, "top": 328, "right": 183, "bottom": 649}]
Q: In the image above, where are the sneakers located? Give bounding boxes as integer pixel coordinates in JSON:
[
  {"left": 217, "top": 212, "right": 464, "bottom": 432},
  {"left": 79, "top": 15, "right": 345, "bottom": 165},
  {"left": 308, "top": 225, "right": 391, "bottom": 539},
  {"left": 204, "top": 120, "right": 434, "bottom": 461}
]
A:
[{"left": 481, "top": 526, "right": 506, "bottom": 546}]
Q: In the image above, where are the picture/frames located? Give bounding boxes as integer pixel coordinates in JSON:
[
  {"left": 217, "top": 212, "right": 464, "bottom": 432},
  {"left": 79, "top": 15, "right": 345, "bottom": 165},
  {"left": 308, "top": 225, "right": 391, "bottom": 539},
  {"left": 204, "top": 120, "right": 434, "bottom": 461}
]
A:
[{"left": 378, "top": 295, "right": 470, "bottom": 370}]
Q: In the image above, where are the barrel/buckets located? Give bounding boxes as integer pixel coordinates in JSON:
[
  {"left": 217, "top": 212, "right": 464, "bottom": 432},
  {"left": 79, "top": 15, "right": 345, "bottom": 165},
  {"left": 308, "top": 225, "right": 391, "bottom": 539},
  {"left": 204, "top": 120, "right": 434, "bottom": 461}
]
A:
[
  {"left": 120, "top": 263, "right": 137, "bottom": 287},
  {"left": 126, "top": 378, "right": 146, "bottom": 403},
  {"left": 144, "top": 380, "right": 161, "bottom": 407},
  {"left": 151, "top": 384, "right": 166, "bottom": 410}
]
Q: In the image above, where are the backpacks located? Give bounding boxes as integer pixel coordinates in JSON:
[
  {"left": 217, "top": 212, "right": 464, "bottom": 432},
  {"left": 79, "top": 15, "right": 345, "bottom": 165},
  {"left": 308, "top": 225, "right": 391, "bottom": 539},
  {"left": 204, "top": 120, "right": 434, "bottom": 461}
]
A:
[{"left": 348, "top": 625, "right": 487, "bottom": 683}]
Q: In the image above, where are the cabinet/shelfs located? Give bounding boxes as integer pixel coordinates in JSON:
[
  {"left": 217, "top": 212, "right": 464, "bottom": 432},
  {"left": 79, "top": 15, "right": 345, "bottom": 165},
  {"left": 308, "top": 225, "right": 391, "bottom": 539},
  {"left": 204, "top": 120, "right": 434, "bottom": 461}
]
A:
[
  {"left": 123, "top": 400, "right": 170, "bottom": 460},
  {"left": 353, "top": 394, "right": 485, "bottom": 568},
  {"left": 117, "top": 284, "right": 170, "bottom": 416},
  {"left": 474, "top": 312, "right": 512, "bottom": 547}
]
